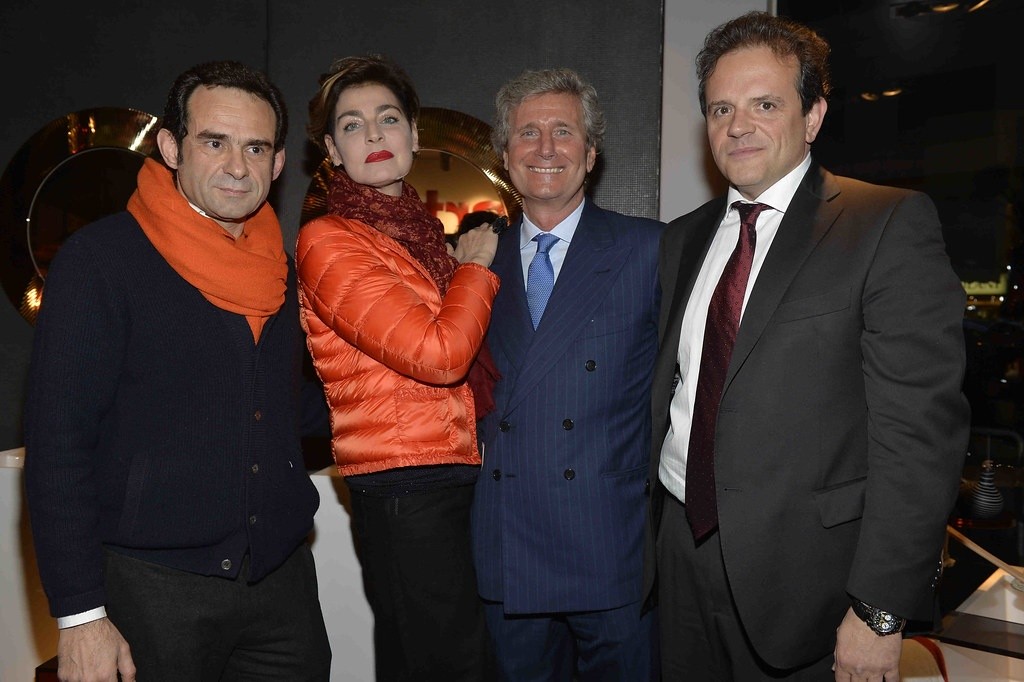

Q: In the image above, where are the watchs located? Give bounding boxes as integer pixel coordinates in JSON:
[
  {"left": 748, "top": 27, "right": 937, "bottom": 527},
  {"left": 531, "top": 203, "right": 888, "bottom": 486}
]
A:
[{"left": 852, "top": 598, "right": 906, "bottom": 636}]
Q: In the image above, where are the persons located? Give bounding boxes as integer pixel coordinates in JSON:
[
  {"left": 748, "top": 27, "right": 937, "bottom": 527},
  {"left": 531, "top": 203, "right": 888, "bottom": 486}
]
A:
[
  {"left": 639, "top": 12, "right": 971, "bottom": 682},
  {"left": 20, "top": 61, "right": 333, "bottom": 682},
  {"left": 469, "top": 65, "right": 667, "bottom": 682},
  {"left": 294, "top": 54, "right": 502, "bottom": 682}
]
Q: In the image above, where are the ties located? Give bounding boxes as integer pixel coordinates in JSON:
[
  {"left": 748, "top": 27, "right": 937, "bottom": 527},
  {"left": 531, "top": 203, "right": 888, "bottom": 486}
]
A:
[
  {"left": 525, "top": 232, "right": 560, "bottom": 332},
  {"left": 685, "top": 201, "right": 774, "bottom": 541}
]
